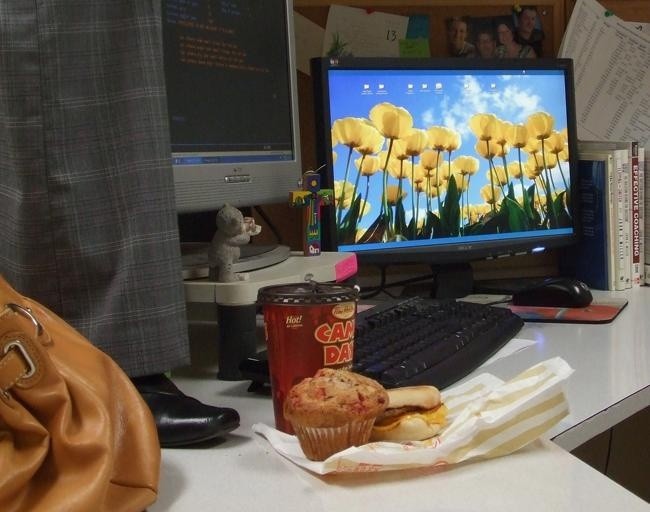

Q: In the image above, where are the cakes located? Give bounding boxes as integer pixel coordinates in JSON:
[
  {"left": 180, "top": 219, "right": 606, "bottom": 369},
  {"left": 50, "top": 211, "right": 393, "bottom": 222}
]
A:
[{"left": 283, "top": 368, "right": 389, "bottom": 461}]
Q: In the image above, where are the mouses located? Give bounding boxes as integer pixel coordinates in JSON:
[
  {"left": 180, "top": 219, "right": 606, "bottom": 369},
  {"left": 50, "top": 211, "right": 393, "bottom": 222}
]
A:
[{"left": 512, "top": 278, "right": 593, "bottom": 308}]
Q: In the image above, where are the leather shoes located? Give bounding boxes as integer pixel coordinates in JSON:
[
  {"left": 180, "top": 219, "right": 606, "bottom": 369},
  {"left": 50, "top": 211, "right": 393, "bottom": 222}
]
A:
[{"left": 127, "top": 374, "right": 239, "bottom": 448}]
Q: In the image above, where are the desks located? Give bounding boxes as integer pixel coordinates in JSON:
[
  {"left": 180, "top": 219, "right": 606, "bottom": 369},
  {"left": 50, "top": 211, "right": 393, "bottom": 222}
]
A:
[{"left": 143, "top": 258, "right": 650, "bottom": 512}]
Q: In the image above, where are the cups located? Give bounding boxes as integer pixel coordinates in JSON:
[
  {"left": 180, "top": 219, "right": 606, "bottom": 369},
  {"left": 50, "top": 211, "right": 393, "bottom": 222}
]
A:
[{"left": 258, "top": 279, "right": 361, "bottom": 436}]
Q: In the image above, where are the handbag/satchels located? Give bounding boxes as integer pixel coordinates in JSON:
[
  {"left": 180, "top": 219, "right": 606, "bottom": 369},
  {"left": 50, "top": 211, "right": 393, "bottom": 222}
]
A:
[{"left": 0, "top": 276, "right": 162, "bottom": 512}]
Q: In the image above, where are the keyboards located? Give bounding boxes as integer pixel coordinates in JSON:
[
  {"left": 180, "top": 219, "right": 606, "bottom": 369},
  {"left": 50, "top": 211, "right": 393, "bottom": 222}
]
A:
[{"left": 237, "top": 295, "right": 524, "bottom": 395}]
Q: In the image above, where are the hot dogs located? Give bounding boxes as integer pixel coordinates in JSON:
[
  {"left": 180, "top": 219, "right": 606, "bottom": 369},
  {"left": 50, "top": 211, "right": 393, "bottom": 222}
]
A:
[{"left": 368, "top": 386, "right": 448, "bottom": 441}]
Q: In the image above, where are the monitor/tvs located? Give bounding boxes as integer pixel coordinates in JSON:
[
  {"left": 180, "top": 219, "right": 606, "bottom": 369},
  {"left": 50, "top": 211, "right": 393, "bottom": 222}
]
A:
[
  {"left": 309, "top": 57, "right": 585, "bottom": 304},
  {"left": 161, "top": 0, "right": 304, "bottom": 280}
]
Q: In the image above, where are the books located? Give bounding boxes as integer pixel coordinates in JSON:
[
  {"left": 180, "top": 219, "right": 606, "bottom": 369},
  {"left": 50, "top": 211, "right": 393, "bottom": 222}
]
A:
[{"left": 574, "top": 135, "right": 649, "bottom": 291}]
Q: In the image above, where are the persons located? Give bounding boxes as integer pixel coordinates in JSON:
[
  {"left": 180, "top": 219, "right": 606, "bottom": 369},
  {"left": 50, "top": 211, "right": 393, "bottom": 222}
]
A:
[
  {"left": 494, "top": 20, "right": 538, "bottom": 58},
  {"left": 513, "top": 5, "right": 554, "bottom": 57},
  {"left": 448, "top": 18, "right": 477, "bottom": 65},
  {"left": 0, "top": 0, "right": 241, "bottom": 450},
  {"left": 477, "top": 31, "right": 494, "bottom": 61}
]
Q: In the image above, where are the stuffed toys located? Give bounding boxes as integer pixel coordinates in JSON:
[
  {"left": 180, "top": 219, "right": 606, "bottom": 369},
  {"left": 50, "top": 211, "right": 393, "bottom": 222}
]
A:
[{"left": 207, "top": 199, "right": 254, "bottom": 284}]
